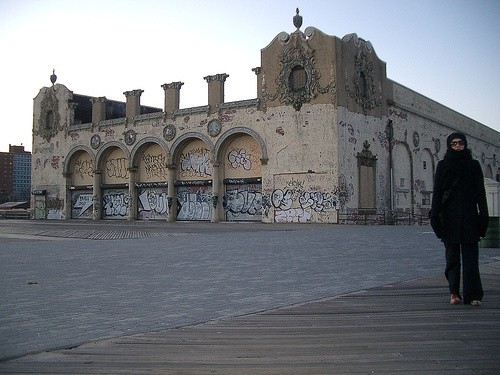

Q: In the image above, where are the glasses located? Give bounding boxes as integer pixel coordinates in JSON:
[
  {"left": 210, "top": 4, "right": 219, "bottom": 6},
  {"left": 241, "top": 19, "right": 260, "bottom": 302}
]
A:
[{"left": 450, "top": 141, "right": 465, "bottom": 146}]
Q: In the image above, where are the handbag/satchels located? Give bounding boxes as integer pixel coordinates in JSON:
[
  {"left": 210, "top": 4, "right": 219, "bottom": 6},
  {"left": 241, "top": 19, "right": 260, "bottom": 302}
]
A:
[{"left": 428, "top": 209, "right": 443, "bottom": 239}]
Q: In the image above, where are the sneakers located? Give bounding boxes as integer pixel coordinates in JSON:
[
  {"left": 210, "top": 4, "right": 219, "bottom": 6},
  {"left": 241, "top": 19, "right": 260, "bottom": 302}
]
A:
[
  {"left": 471, "top": 299, "right": 481, "bottom": 306},
  {"left": 450, "top": 293, "right": 463, "bottom": 304}
]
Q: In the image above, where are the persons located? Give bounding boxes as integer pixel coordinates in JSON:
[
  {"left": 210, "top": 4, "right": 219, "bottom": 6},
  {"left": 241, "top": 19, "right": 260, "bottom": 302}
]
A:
[{"left": 429, "top": 132, "right": 489, "bottom": 306}]
